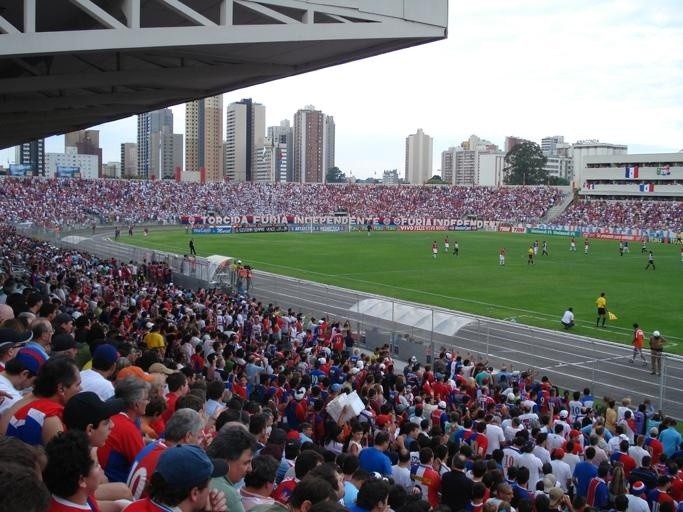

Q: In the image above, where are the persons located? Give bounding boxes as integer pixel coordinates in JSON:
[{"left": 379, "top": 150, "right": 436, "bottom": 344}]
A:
[
  {"left": 350, "top": 181, "right": 683, "bottom": 267},
  {"left": 350, "top": 350, "right": 682, "bottom": 511},
  {"left": 1, "top": 171, "right": 349, "bottom": 510},
  {"left": 648, "top": 329, "right": 667, "bottom": 377},
  {"left": 628, "top": 323, "right": 646, "bottom": 366},
  {"left": 559, "top": 307, "right": 575, "bottom": 330},
  {"left": 593, "top": 291, "right": 610, "bottom": 328}
]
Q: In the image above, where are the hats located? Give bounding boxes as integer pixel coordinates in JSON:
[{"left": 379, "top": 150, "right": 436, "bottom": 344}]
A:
[
  {"left": 0, "top": 317, "right": 33, "bottom": 354},
  {"left": 62, "top": 392, "right": 125, "bottom": 429},
  {"left": 15, "top": 341, "right": 48, "bottom": 376},
  {"left": 154, "top": 442, "right": 229, "bottom": 491},
  {"left": 115, "top": 365, "right": 155, "bottom": 382},
  {"left": 91, "top": 343, "right": 121, "bottom": 368}
]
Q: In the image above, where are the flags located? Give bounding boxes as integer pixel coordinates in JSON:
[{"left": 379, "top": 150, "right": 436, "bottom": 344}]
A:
[{"left": 608, "top": 310, "right": 618, "bottom": 321}]
